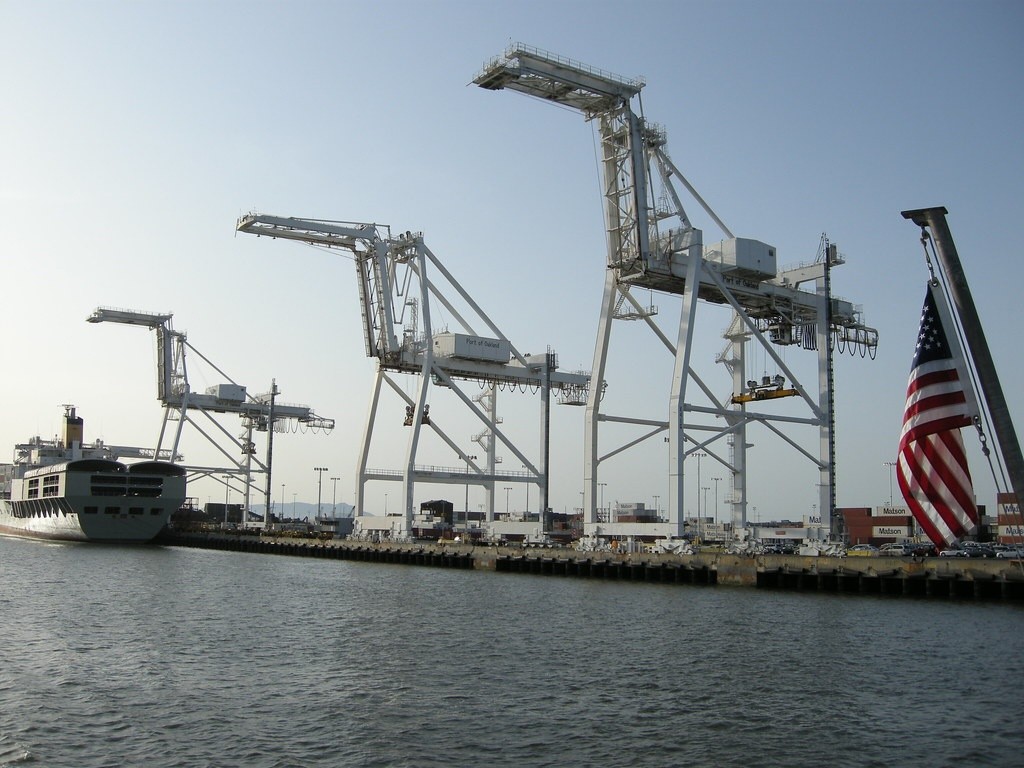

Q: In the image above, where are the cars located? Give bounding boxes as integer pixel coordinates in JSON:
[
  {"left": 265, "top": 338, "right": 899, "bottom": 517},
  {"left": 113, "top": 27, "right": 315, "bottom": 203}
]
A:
[{"left": 749, "top": 541, "right": 1024, "bottom": 558}]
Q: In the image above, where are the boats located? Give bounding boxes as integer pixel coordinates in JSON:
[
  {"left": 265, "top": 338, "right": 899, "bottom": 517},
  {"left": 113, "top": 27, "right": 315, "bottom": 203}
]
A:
[{"left": 0, "top": 439, "right": 188, "bottom": 545}]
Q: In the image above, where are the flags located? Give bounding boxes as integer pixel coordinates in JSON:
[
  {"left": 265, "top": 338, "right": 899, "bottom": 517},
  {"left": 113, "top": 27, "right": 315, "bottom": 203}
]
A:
[{"left": 896, "top": 277, "right": 983, "bottom": 552}]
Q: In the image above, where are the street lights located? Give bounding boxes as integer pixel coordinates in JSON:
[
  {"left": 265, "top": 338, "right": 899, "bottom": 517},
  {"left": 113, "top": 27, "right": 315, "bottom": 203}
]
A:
[
  {"left": 314, "top": 468, "right": 329, "bottom": 535},
  {"left": 651, "top": 495, "right": 660, "bottom": 511},
  {"left": 521, "top": 464, "right": 535, "bottom": 511},
  {"left": 883, "top": 462, "right": 899, "bottom": 507},
  {"left": 221, "top": 474, "right": 235, "bottom": 531},
  {"left": 701, "top": 487, "right": 710, "bottom": 526},
  {"left": 504, "top": 488, "right": 512, "bottom": 519},
  {"left": 691, "top": 452, "right": 708, "bottom": 540},
  {"left": 458, "top": 455, "right": 478, "bottom": 527},
  {"left": 384, "top": 494, "right": 387, "bottom": 516},
  {"left": 599, "top": 482, "right": 608, "bottom": 517},
  {"left": 282, "top": 484, "right": 286, "bottom": 517},
  {"left": 710, "top": 477, "right": 723, "bottom": 532},
  {"left": 329, "top": 477, "right": 341, "bottom": 518},
  {"left": 292, "top": 493, "right": 297, "bottom": 516}
]
